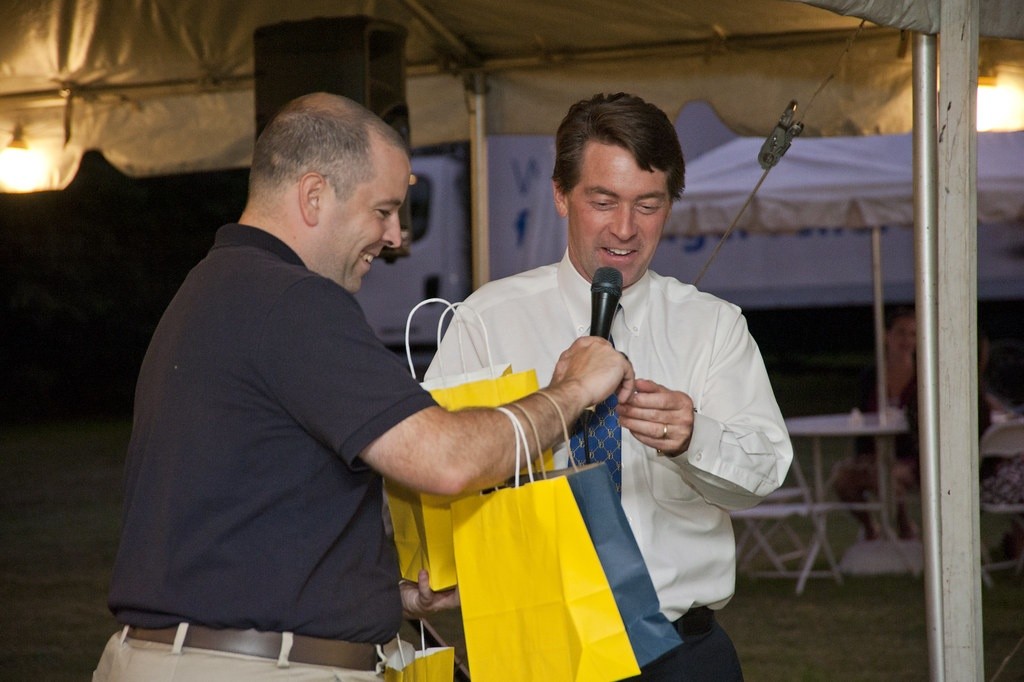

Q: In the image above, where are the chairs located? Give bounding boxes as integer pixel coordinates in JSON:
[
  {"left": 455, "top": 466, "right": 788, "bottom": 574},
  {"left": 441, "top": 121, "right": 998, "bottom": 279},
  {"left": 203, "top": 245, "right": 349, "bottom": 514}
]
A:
[
  {"left": 730, "top": 457, "right": 845, "bottom": 587},
  {"left": 979, "top": 420, "right": 1024, "bottom": 589}
]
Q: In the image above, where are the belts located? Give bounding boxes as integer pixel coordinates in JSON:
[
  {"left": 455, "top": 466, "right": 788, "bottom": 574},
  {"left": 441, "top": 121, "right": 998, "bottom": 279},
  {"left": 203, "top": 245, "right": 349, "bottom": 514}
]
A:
[{"left": 120, "top": 621, "right": 385, "bottom": 676}]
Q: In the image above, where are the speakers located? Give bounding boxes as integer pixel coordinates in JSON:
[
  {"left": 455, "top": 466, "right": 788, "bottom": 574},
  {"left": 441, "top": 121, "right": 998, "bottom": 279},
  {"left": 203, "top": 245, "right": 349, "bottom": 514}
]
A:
[{"left": 254, "top": 13, "right": 413, "bottom": 264}]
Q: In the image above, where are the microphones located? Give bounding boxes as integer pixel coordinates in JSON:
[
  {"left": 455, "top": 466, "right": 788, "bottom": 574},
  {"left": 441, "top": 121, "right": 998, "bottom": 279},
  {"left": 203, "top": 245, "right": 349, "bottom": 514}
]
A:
[{"left": 583, "top": 266, "right": 622, "bottom": 425}]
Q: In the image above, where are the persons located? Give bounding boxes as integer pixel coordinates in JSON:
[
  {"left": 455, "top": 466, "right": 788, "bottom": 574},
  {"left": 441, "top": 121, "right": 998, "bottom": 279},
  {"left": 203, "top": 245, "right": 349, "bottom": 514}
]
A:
[
  {"left": 832, "top": 306, "right": 928, "bottom": 541},
  {"left": 417, "top": 90, "right": 794, "bottom": 682},
  {"left": 90, "top": 93, "right": 636, "bottom": 681}
]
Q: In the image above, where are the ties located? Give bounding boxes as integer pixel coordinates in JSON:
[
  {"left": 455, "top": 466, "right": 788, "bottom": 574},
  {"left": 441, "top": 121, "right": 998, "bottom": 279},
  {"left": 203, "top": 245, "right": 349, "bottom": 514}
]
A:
[{"left": 567, "top": 303, "right": 619, "bottom": 500}]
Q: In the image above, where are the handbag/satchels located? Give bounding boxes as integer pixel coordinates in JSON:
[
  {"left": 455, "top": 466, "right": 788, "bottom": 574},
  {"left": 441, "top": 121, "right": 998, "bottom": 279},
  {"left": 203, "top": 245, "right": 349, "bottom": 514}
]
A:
[
  {"left": 449, "top": 391, "right": 686, "bottom": 682},
  {"left": 387, "top": 297, "right": 555, "bottom": 592},
  {"left": 373, "top": 618, "right": 454, "bottom": 682}
]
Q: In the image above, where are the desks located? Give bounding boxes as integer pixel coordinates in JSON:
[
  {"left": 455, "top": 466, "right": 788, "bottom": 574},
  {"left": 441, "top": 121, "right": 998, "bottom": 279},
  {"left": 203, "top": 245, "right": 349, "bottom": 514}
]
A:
[{"left": 786, "top": 410, "right": 922, "bottom": 595}]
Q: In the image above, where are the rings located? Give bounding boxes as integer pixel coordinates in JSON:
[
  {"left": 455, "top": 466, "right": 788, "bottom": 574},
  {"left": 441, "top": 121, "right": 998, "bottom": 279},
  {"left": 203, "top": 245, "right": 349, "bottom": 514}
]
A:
[{"left": 662, "top": 425, "right": 669, "bottom": 437}]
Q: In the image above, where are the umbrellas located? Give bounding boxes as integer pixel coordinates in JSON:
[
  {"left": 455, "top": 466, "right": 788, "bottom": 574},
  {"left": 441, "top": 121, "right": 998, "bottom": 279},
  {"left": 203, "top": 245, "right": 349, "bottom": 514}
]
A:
[{"left": 658, "top": 135, "right": 1024, "bottom": 542}]
